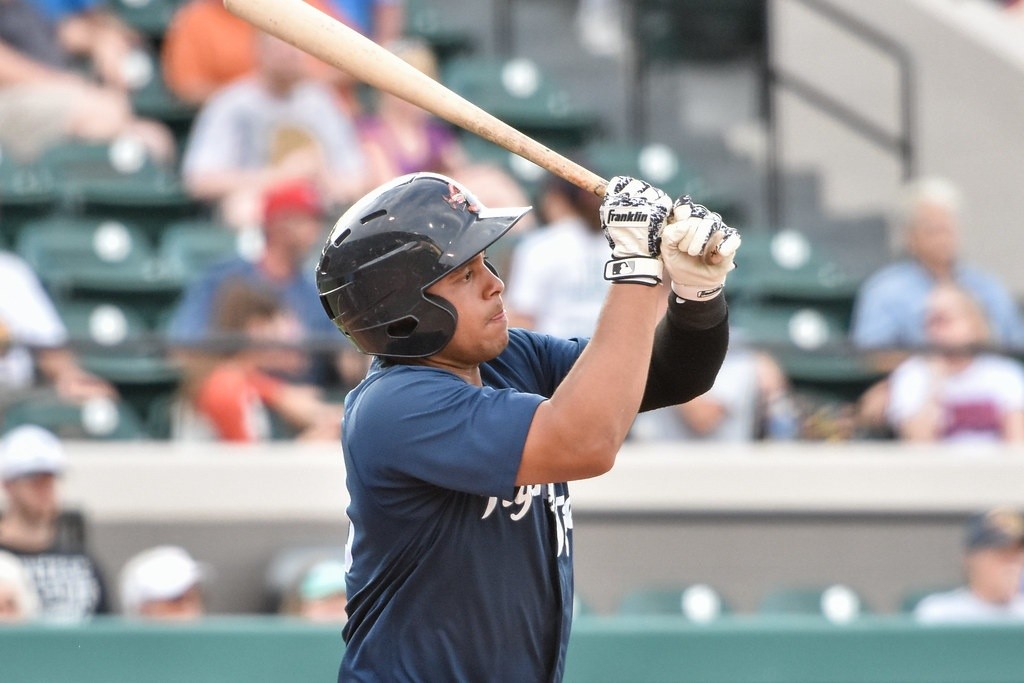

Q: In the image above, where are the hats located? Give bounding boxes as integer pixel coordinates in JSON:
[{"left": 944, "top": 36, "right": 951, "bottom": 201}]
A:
[
  {"left": 117, "top": 543, "right": 201, "bottom": 601},
  {"left": 967, "top": 505, "right": 1022, "bottom": 546},
  {"left": 0, "top": 425, "right": 80, "bottom": 477},
  {"left": 258, "top": 179, "right": 326, "bottom": 219}
]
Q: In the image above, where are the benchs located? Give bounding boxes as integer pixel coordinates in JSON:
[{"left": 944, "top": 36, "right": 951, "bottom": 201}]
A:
[{"left": 0, "top": 0, "right": 1024, "bottom": 683}]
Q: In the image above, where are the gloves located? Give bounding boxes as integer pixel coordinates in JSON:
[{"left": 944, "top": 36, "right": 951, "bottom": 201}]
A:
[
  {"left": 658, "top": 194, "right": 741, "bottom": 302},
  {"left": 598, "top": 175, "right": 674, "bottom": 286}
]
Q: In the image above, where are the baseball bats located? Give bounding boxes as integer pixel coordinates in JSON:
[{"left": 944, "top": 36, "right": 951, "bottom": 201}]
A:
[{"left": 223, "top": 0, "right": 727, "bottom": 266}]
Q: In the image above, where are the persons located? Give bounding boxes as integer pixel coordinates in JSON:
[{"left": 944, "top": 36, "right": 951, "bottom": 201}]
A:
[
  {"left": 316, "top": 173, "right": 741, "bottom": 683},
  {"left": 0, "top": 1, "right": 1022, "bottom": 622}
]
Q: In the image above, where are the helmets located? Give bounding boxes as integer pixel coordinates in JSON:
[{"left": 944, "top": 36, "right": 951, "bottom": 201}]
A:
[{"left": 316, "top": 171, "right": 534, "bottom": 357}]
